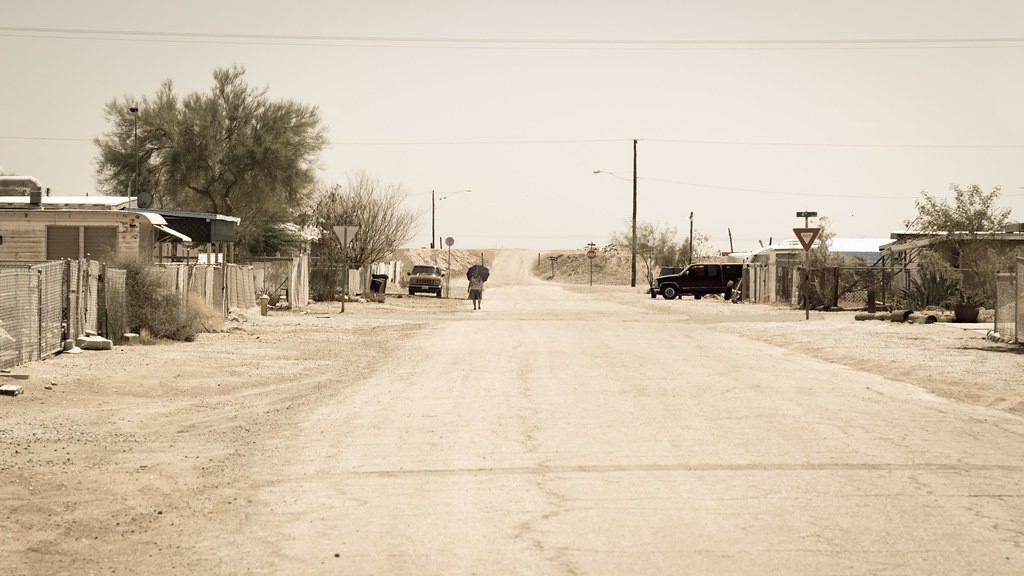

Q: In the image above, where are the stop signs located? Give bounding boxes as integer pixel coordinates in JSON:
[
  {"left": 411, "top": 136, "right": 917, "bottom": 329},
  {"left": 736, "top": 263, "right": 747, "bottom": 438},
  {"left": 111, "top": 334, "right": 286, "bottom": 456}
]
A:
[{"left": 587, "top": 251, "right": 596, "bottom": 259}]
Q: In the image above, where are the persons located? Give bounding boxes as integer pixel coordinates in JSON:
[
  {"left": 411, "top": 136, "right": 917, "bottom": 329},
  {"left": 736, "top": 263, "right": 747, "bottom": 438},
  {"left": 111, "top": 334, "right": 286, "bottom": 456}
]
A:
[{"left": 467, "top": 270, "right": 485, "bottom": 310}]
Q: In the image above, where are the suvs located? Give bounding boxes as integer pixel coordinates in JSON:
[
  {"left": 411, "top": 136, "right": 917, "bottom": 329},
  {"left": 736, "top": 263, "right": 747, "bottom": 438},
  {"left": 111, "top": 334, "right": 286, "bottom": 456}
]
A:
[
  {"left": 407, "top": 265, "right": 447, "bottom": 297},
  {"left": 654, "top": 263, "right": 742, "bottom": 300},
  {"left": 650, "top": 267, "right": 685, "bottom": 298}
]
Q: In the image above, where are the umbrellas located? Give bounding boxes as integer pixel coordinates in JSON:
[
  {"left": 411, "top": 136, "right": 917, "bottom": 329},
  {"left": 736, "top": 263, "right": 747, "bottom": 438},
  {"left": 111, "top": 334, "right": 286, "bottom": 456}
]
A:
[{"left": 466, "top": 264, "right": 490, "bottom": 282}]
